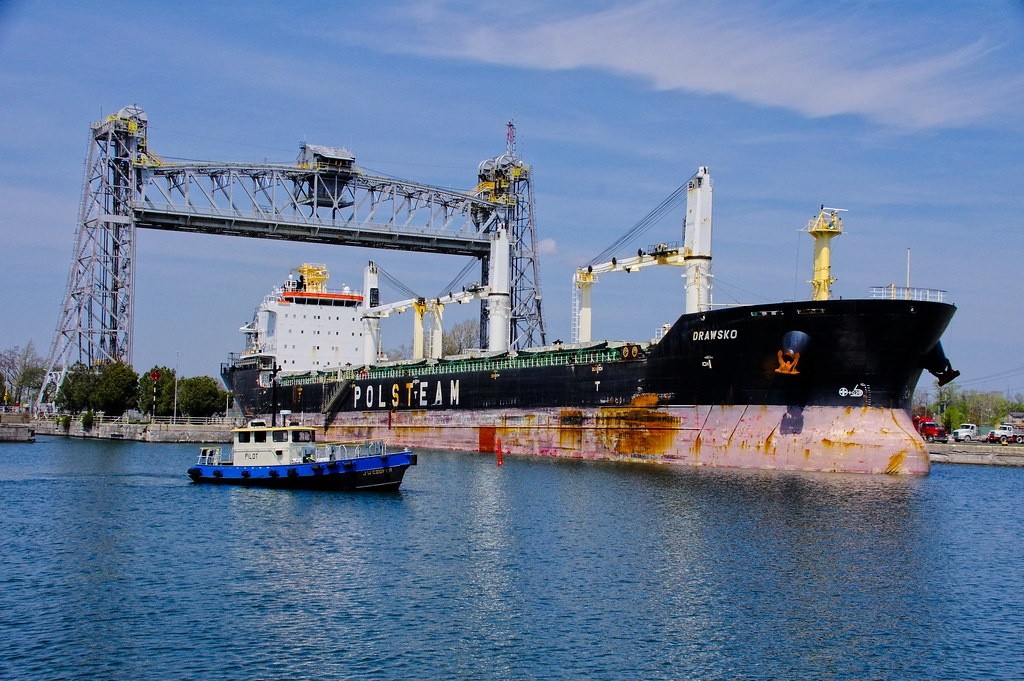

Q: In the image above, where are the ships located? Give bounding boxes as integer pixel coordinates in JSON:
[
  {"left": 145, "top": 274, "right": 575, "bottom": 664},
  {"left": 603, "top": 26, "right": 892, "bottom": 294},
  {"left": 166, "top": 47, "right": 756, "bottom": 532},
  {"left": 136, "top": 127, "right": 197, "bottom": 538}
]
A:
[{"left": 219, "top": 165, "right": 961, "bottom": 476}]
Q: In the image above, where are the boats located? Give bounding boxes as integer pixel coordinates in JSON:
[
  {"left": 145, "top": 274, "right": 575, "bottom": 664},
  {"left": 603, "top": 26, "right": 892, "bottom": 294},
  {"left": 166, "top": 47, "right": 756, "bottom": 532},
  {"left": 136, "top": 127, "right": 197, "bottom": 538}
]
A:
[
  {"left": 515, "top": 339, "right": 608, "bottom": 359},
  {"left": 437, "top": 349, "right": 510, "bottom": 364},
  {"left": 280, "top": 371, "right": 312, "bottom": 380},
  {"left": 317, "top": 363, "right": 365, "bottom": 376},
  {"left": 369, "top": 359, "right": 428, "bottom": 369},
  {"left": 187, "top": 362, "right": 417, "bottom": 493}
]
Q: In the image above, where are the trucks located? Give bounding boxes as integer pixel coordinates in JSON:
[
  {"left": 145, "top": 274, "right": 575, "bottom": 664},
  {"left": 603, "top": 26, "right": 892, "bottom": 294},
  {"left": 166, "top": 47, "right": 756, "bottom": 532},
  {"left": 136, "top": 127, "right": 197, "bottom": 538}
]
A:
[
  {"left": 951, "top": 423, "right": 988, "bottom": 443},
  {"left": 912, "top": 416, "right": 949, "bottom": 443},
  {"left": 988, "top": 422, "right": 1024, "bottom": 443}
]
